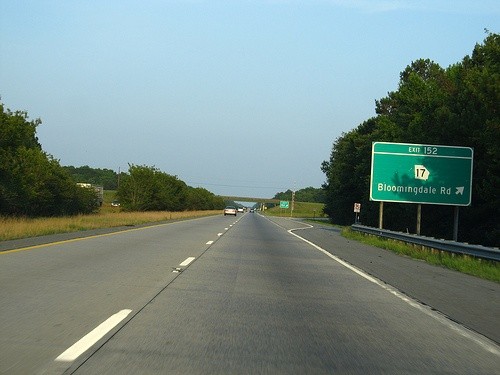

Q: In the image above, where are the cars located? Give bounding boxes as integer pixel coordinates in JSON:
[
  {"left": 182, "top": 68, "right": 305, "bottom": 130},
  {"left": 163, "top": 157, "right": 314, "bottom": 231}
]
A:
[
  {"left": 224, "top": 206, "right": 237, "bottom": 216},
  {"left": 111, "top": 200, "right": 120, "bottom": 207},
  {"left": 238, "top": 207, "right": 256, "bottom": 213}
]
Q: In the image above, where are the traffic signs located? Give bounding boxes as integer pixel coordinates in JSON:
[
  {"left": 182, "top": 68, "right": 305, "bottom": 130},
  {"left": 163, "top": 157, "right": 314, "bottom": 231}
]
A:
[{"left": 369, "top": 141, "right": 474, "bottom": 206}]
